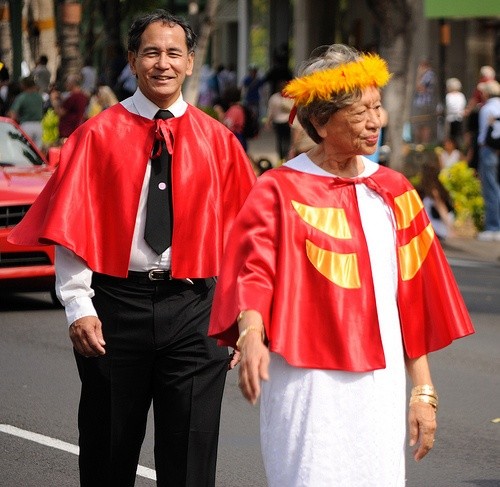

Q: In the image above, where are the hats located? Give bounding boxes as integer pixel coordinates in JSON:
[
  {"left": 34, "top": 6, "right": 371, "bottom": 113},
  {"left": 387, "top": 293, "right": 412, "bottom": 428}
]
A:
[
  {"left": 248, "top": 62, "right": 258, "bottom": 70},
  {"left": 477, "top": 80, "right": 500, "bottom": 96},
  {"left": 37, "top": 54, "right": 48, "bottom": 64}
]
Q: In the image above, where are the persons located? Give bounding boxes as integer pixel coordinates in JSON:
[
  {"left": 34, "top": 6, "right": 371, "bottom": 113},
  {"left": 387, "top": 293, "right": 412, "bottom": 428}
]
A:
[
  {"left": 207, "top": 46, "right": 475, "bottom": 487},
  {"left": 6, "top": 8, "right": 257, "bottom": 486},
  {"left": 180, "top": 52, "right": 320, "bottom": 173},
  {"left": 1, "top": 48, "right": 139, "bottom": 165},
  {"left": 420, "top": 65, "right": 499, "bottom": 247}
]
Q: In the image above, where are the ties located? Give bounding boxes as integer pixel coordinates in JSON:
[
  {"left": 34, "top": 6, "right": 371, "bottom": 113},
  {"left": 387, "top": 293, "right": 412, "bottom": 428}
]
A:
[{"left": 144, "top": 109, "right": 172, "bottom": 255}]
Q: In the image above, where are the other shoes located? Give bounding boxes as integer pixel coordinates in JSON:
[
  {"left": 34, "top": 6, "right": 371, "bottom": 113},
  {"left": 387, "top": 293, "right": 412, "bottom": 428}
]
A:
[{"left": 477, "top": 230, "right": 500, "bottom": 241}]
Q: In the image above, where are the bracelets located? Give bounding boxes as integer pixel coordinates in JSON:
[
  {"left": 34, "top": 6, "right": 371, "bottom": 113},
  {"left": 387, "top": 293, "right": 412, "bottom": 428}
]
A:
[
  {"left": 235, "top": 326, "right": 264, "bottom": 349},
  {"left": 409, "top": 385, "right": 439, "bottom": 410}
]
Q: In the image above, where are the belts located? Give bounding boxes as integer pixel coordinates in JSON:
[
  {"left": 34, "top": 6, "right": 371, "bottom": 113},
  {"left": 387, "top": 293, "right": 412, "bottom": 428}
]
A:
[{"left": 124, "top": 267, "right": 172, "bottom": 282}]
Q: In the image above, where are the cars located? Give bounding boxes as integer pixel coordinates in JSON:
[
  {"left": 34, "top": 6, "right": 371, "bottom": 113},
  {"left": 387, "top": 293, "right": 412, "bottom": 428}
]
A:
[{"left": 0, "top": 116, "right": 64, "bottom": 310}]
